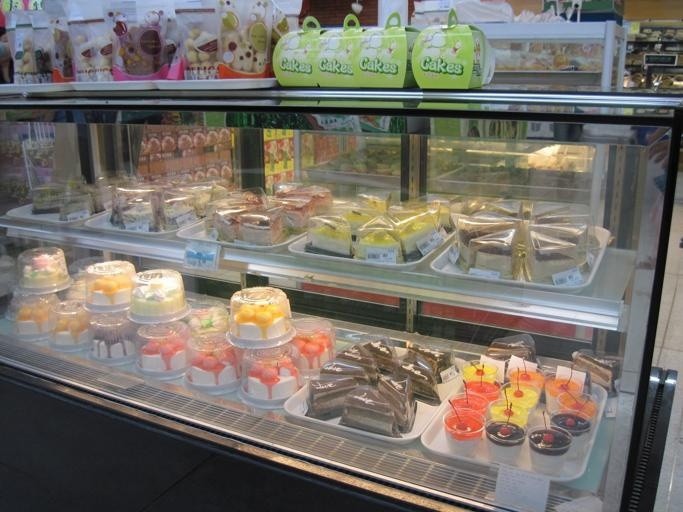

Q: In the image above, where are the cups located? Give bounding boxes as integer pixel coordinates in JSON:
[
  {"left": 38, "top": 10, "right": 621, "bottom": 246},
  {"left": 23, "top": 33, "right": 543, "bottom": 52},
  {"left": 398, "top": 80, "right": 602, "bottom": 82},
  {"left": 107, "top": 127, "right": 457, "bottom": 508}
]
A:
[{"left": 442, "top": 358, "right": 598, "bottom": 478}]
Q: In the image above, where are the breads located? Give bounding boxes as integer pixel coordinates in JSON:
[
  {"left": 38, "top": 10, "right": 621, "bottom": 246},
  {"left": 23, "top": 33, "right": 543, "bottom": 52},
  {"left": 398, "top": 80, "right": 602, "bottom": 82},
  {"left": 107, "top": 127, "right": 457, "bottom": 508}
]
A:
[{"left": 327, "top": 147, "right": 400, "bottom": 174}]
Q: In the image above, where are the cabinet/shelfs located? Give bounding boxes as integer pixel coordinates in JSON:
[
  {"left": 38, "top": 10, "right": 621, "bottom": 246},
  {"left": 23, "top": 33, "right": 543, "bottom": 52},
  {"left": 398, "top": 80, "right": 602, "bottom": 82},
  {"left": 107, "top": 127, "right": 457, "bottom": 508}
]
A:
[{"left": 0, "top": 0, "right": 683, "bottom": 512}]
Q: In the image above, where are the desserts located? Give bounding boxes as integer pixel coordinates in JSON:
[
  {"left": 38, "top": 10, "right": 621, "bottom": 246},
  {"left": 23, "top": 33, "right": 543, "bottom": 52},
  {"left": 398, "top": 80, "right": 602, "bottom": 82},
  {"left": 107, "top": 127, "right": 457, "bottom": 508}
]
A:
[
  {"left": 27, "top": 176, "right": 603, "bottom": 288},
  {"left": 9, "top": 245, "right": 617, "bottom": 472}
]
